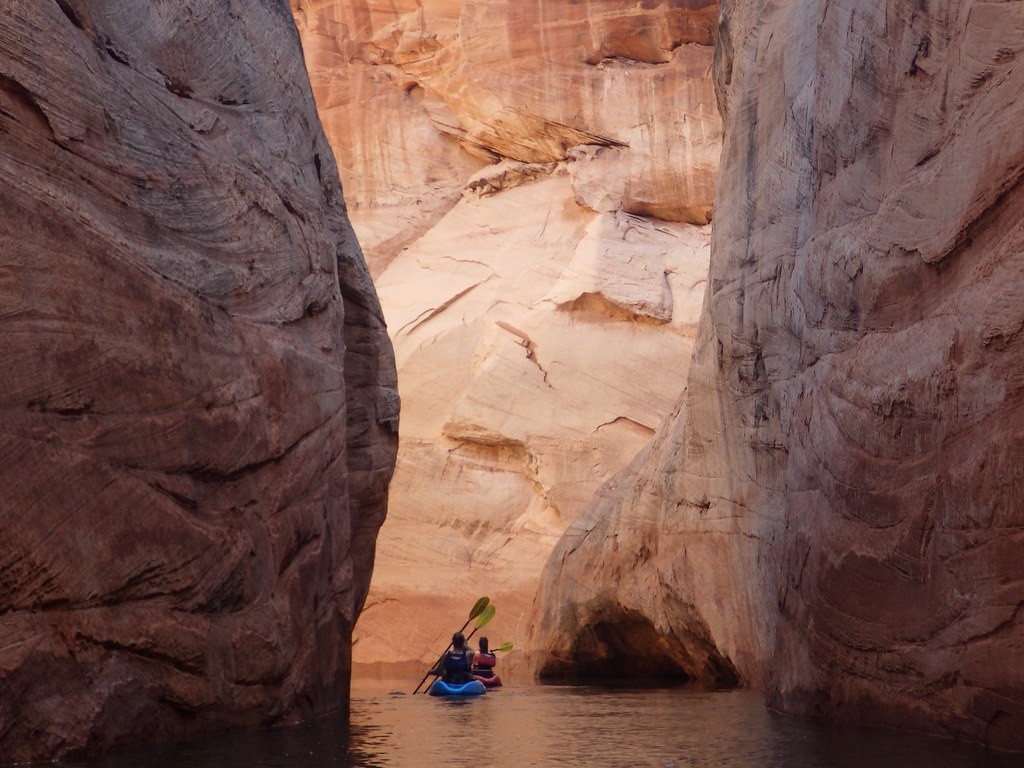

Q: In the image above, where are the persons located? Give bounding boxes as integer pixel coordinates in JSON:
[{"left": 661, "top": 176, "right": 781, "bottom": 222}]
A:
[
  {"left": 426, "top": 630, "right": 475, "bottom": 684},
  {"left": 471, "top": 636, "right": 496, "bottom": 670}
]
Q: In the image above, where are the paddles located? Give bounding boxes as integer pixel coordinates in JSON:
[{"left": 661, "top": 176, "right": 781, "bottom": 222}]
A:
[
  {"left": 412, "top": 596, "right": 490, "bottom": 696},
  {"left": 490, "top": 642, "right": 514, "bottom": 654},
  {"left": 423, "top": 604, "right": 496, "bottom": 695}
]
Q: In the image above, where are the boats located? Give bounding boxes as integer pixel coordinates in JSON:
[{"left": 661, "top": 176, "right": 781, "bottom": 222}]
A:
[
  {"left": 470, "top": 669, "right": 503, "bottom": 687},
  {"left": 428, "top": 679, "right": 487, "bottom": 697}
]
